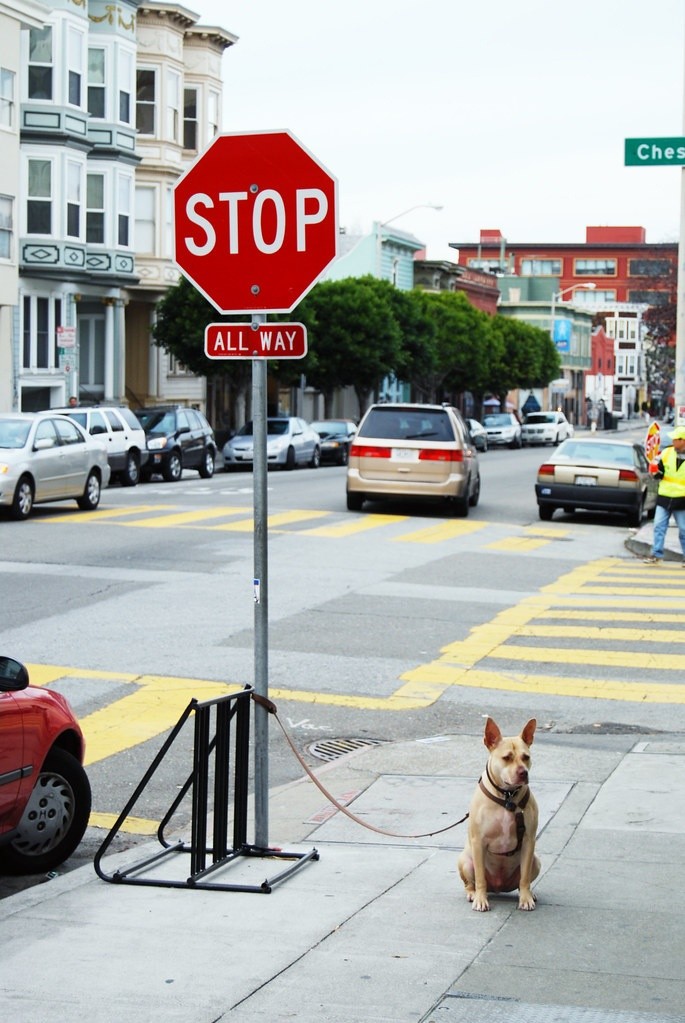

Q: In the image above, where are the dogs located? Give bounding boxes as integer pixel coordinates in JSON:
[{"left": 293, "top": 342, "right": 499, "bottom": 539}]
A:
[{"left": 458, "top": 717, "right": 542, "bottom": 911}]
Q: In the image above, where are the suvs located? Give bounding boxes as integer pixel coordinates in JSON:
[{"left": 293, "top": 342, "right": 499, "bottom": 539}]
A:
[
  {"left": 38, "top": 404, "right": 149, "bottom": 487},
  {"left": 347, "top": 401, "right": 481, "bottom": 517}
]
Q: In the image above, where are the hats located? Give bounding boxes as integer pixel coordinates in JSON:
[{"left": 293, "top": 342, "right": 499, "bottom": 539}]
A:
[{"left": 668, "top": 427, "right": 685, "bottom": 440}]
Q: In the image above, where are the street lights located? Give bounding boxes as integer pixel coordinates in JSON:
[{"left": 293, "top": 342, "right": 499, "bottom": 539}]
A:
[
  {"left": 547, "top": 283, "right": 596, "bottom": 411},
  {"left": 376, "top": 203, "right": 442, "bottom": 280}
]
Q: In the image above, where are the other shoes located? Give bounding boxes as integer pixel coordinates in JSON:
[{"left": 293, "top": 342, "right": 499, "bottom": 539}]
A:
[
  {"left": 682, "top": 561, "right": 685, "bottom": 567},
  {"left": 643, "top": 555, "right": 664, "bottom": 564}
]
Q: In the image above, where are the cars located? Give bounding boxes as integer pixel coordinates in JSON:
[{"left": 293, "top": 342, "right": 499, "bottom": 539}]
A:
[
  {"left": 310, "top": 419, "right": 358, "bottom": 467},
  {"left": 0, "top": 656, "right": 93, "bottom": 875},
  {"left": 535, "top": 438, "right": 662, "bottom": 527},
  {"left": 223, "top": 418, "right": 320, "bottom": 472},
  {"left": 0, "top": 413, "right": 111, "bottom": 520},
  {"left": 133, "top": 404, "right": 217, "bottom": 482},
  {"left": 522, "top": 411, "right": 574, "bottom": 446},
  {"left": 481, "top": 413, "right": 522, "bottom": 449},
  {"left": 465, "top": 419, "right": 488, "bottom": 452}
]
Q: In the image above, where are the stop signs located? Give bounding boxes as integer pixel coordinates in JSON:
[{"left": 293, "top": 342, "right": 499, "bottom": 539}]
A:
[{"left": 172, "top": 129, "right": 336, "bottom": 313}]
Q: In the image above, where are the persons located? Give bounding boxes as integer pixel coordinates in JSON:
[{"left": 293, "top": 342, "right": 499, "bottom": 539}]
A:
[
  {"left": 69, "top": 397, "right": 77, "bottom": 407},
  {"left": 643, "top": 427, "right": 685, "bottom": 567}
]
